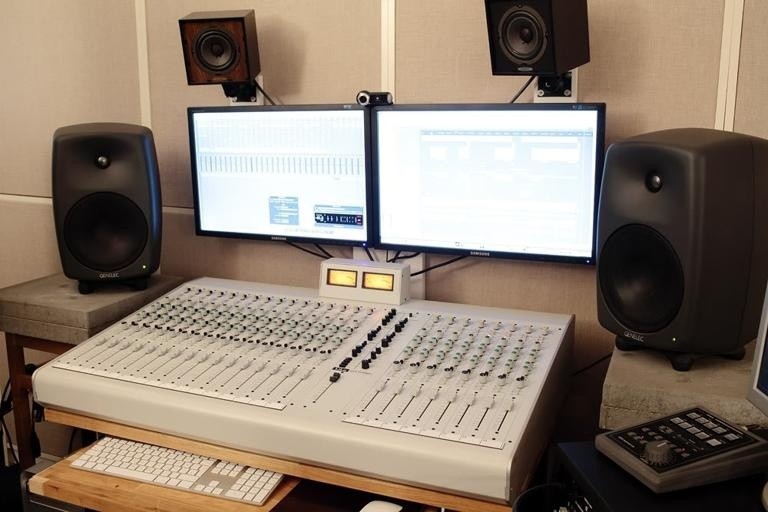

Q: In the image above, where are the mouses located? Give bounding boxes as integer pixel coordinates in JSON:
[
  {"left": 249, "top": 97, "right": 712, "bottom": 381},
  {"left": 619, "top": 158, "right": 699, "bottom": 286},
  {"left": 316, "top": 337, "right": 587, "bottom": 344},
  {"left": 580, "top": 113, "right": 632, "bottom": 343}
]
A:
[{"left": 360, "top": 498, "right": 403, "bottom": 512}]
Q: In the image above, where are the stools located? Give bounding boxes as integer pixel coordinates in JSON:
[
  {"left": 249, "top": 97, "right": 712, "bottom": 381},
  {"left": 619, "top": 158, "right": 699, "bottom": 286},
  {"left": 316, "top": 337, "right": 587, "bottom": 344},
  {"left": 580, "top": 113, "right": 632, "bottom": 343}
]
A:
[{"left": 1, "top": 275, "right": 186, "bottom": 471}]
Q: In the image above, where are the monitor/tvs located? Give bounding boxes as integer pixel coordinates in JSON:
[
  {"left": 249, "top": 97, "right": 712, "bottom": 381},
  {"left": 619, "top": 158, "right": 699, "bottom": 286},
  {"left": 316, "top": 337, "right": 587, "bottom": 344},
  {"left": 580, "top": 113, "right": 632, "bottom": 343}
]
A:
[
  {"left": 186, "top": 103, "right": 372, "bottom": 248},
  {"left": 370, "top": 103, "right": 606, "bottom": 267}
]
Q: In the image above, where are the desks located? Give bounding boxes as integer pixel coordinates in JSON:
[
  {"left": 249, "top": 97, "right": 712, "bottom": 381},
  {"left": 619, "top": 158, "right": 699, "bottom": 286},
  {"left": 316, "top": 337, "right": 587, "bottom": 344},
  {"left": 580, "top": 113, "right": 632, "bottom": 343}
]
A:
[{"left": 26, "top": 404, "right": 526, "bottom": 512}]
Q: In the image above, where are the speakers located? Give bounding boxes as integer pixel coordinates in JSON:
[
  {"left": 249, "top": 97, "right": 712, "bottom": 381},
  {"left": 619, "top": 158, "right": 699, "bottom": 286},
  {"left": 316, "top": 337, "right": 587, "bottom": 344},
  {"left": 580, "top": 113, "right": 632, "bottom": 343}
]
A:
[
  {"left": 480, "top": 0, "right": 591, "bottom": 80},
  {"left": 596, "top": 126, "right": 768, "bottom": 371},
  {"left": 177, "top": 9, "right": 264, "bottom": 87},
  {"left": 52, "top": 122, "right": 163, "bottom": 293}
]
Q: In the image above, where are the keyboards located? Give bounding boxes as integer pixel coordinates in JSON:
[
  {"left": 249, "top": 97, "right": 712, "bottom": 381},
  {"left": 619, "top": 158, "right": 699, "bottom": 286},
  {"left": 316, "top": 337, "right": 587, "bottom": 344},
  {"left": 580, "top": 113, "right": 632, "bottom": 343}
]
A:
[{"left": 70, "top": 434, "right": 285, "bottom": 505}]
